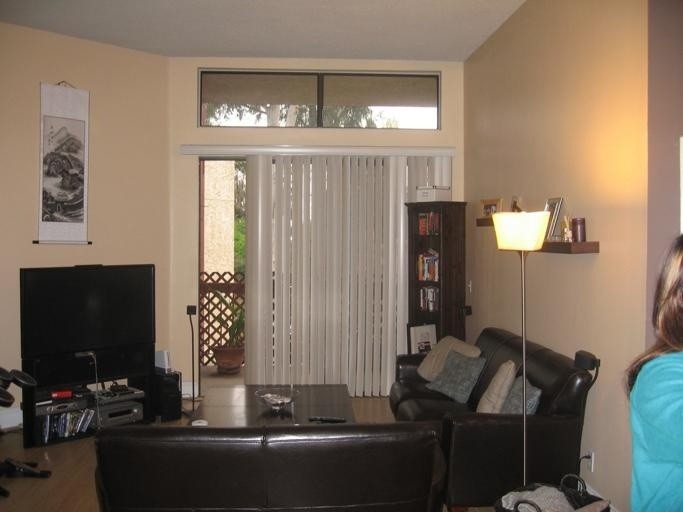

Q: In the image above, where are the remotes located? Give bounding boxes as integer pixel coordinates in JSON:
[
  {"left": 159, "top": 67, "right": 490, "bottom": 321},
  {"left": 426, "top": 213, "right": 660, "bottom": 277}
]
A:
[{"left": 309, "top": 416, "right": 346, "bottom": 423}]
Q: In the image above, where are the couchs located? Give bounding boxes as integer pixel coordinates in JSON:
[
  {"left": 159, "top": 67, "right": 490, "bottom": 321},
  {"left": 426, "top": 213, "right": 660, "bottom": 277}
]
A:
[
  {"left": 388, "top": 326, "right": 592, "bottom": 507},
  {"left": 90, "top": 422, "right": 446, "bottom": 511}
]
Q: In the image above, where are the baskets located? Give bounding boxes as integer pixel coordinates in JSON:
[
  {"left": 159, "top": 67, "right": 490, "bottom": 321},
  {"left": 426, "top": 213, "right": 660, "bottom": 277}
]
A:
[{"left": 493, "top": 472, "right": 611, "bottom": 512}]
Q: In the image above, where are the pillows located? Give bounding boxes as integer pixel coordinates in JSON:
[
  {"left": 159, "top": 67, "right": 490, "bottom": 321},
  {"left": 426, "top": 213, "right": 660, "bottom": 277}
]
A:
[
  {"left": 416, "top": 335, "right": 481, "bottom": 382},
  {"left": 501, "top": 372, "right": 543, "bottom": 416},
  {"left": 474, "top": 359, "right": 516, "bottom": 417},
  {"left": 424, "top": 349, "right": 488, "bottom": 405}
]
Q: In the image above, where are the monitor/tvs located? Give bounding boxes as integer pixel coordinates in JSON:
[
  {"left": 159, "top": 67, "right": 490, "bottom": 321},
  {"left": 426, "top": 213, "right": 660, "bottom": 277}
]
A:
[{"left": 20, "top": 264, "right": 156, "bottom": 357}]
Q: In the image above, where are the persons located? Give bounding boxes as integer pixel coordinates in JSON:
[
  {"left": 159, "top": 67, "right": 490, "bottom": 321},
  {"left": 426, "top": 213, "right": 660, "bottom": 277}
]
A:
[{"left": 627, "top": 233, "right": 683, "bottom": 512}]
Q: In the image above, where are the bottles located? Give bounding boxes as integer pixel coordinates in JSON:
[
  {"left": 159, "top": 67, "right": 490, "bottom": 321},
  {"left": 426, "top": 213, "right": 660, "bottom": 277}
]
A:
[{"left": 571, "top": 218, "right": 586, "bottom": 243}]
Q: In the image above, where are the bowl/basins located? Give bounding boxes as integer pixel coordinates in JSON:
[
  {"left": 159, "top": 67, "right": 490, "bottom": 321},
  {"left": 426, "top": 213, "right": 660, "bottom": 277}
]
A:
[{"left": 254, "top": 387, "right": 300, "bottom": 412}]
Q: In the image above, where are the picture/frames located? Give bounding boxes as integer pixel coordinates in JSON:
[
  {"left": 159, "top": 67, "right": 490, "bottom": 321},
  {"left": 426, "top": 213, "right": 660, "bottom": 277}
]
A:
[
  {"left": 542, "top": 196, "right": 562, "bottom": 241},
  {"left": 407, "top": 321, "right": 437, "bottom": 355},
  {"left": 479, "top": 198, "right": 501, "bottom": 219}
]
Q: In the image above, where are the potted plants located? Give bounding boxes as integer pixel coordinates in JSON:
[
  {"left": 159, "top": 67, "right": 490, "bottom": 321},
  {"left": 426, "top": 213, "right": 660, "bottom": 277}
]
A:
[{"left": 207, "top": 287, "right": 244, "bottom": 373}]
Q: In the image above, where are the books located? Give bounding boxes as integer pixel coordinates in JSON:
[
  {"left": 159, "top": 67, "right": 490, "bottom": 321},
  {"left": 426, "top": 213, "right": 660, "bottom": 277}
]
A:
[
  {"left": 44, "top": 406, "right": 95, "bottom": 444},
  {"left": 416, "top": 285, "right": 441, "bottom": 313},
  {"left": 418, "top": 211, "right": 441, "bottom": 235},
  {"left": 416, "top": 248, "right": 439, "bottom": 284}
]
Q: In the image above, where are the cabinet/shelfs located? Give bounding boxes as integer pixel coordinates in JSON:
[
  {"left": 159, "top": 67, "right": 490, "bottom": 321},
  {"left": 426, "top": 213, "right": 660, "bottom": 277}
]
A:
[
  {"left": 403, "top": 201, "right": 468, "bottom": 342},
  {"left": 19, "top": 370, "right": 183, "bottom": 449}
]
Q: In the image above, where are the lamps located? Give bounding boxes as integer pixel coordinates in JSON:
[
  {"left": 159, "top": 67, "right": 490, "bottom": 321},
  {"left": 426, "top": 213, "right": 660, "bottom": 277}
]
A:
[{"left": 491, "top": 211, "right": 552, "bottom": 486}]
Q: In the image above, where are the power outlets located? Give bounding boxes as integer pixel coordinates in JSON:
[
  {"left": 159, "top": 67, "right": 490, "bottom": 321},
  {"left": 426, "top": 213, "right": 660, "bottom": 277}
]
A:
[{"left": 585, "top": 449, "right": 593, "bottom": 472}]
